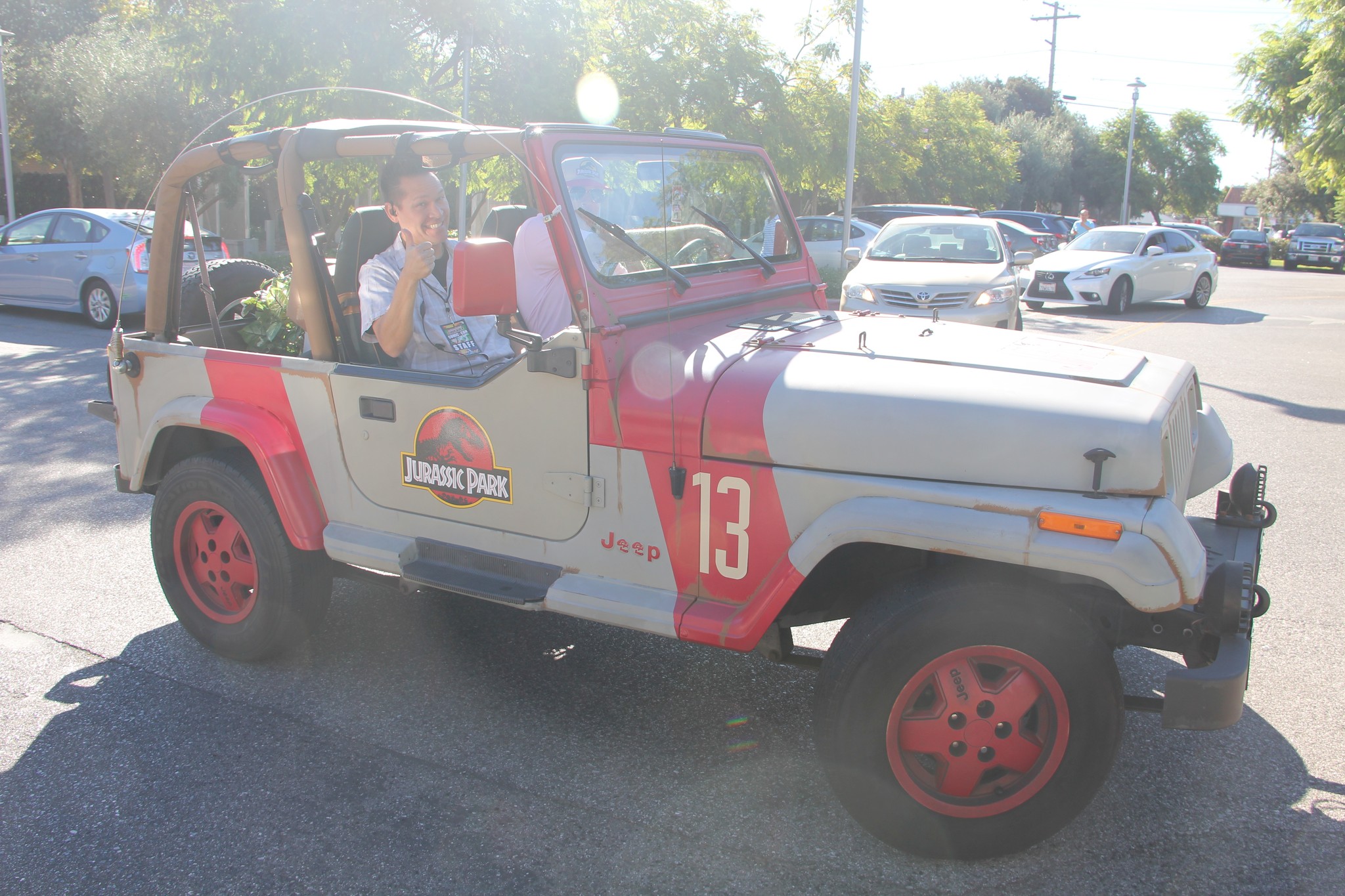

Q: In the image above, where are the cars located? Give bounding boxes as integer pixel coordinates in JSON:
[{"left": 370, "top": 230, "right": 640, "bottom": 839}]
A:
[
  {"left": 725, "top": 215, "right": 882, "bottom": 271},
  {"left": 1278, "top": 222, "right": 1345, "bottom": 274},
  {"left": 1130, "top": 220, "right": 1223, "bottom": 249},
  {"left": 800, "top": 205, "right": 980, "bottom": 242},
  {"left": 840, "top": 217, "right": 1036, "bottom": 334},
  {"left": 979, "top": 210, "right": 1102, "bottom": 253},
  {"left": 1018, "top": 225, "right": 1220, "bottom": 314},
  {"left": 0, "top": 207, "right": 233, "bottom": 329},
  {"left": 1219, "top": 229, "right": 1272, "bottom": 267}
]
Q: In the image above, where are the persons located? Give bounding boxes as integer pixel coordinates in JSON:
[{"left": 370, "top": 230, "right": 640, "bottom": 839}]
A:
[
  {"left": 759, "top": 197, "right": 789, "bottom": 257},
  {"left": 357, "top": 153, "right": 515, "bottom": 381},
  {"left": 513, "top": 155, "right": 734, "bottom": 341},
  {"left": 1070, "top": 209, "right": 1095, "bottom": 249}
]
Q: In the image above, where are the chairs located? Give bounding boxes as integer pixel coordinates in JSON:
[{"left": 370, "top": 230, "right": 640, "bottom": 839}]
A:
[
  {"left": 336, "top": 207, "right": 403, "bottom": 365},
  {"left": 963, "top": 236, "right": 988, "bottom": 260},
  {"left": 902, "top": 235, "right": 924, "bottom": 257},
  {"left": 70, "top": 222, "right": 86, "bottom": 243},
  {"left": 479, "top": 204, "right": 539, "bottom": 246},
  {"left": 1148, "top": 237, "right": 1158, "bottom": 247}
]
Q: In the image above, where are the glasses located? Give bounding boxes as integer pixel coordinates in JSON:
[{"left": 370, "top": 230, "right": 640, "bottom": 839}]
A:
[{"left": 568, "top": 186, "right": 605, "bottom": 203}]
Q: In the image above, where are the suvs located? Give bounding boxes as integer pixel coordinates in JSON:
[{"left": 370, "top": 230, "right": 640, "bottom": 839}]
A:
[{"left": 88, "top": 86, "right": 1278, "bottom": 861}]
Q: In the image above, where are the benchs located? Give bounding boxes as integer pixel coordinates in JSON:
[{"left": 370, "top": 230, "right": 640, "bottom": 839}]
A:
[{"left": 921, "top": 235, "right": 962, "bottom": 258}]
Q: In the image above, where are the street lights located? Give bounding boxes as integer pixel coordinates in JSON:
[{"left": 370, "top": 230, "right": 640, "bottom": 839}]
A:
[{"left": 1119, "top": 75, "right": 1148, "bottom": 222}]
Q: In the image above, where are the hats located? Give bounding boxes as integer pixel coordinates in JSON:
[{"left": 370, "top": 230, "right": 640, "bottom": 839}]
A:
[{"left": 561, "top": 157, "right": 613, "bottom": 190}]
